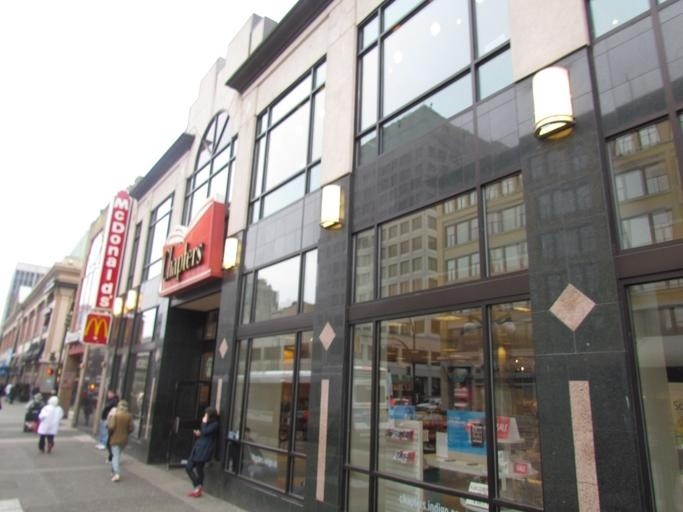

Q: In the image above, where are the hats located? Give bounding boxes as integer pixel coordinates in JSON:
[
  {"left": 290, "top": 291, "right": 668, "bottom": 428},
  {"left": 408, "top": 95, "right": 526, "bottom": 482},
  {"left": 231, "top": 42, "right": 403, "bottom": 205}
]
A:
[{"left": 34, "top": 393, "right": 43, "bottom": 400}]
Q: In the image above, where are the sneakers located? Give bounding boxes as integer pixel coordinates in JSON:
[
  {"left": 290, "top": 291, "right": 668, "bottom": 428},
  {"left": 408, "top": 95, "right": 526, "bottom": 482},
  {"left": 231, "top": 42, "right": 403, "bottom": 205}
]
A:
[
  {"left": 110, "top": 472, "right": 120, "bottom": 481},
  {"left": 188, "top": 484, "right": 202, "bottom": 498},
  {"left": 95, "top": 444, "right": 105, "bottom": 451}
]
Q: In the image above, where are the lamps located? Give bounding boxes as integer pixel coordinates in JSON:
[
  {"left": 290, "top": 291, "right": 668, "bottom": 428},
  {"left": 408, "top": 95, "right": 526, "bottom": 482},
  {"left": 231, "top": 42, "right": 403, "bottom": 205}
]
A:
[
  {"left": 223, "top": 237, "right": 241, "bottom": 272},
  {"left": 112, "top": 289, "right": 138, "bottom": 317},
  {"left": 319, "top": 184, "right": 345, "bottom": 230},
  {"left": 532, "top": 66, "right": 577, "bottom": 140}
]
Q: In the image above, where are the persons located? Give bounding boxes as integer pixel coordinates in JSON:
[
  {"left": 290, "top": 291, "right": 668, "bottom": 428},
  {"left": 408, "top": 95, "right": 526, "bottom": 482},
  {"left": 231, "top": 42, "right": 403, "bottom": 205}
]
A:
[
  {"left": 0, "top": 381, "right": 99, "bottom": 426},
  {"left": 93, "top": 386, "right": 119, "bottom": 450},
  {"left": 104, "top": 398, "right": 135, "bottom": 482},
  {"left": 184, "top": 406, "right": 219, "bottom": 498},
  {"left": 35, "top": 394, "right": 64, "bottom": 453},
  {"left": 243, "top": 426, "right": 265, "bottom": 462},
  {"left": 22, "top": 392, "right": 44, "bottom": 432}
]
